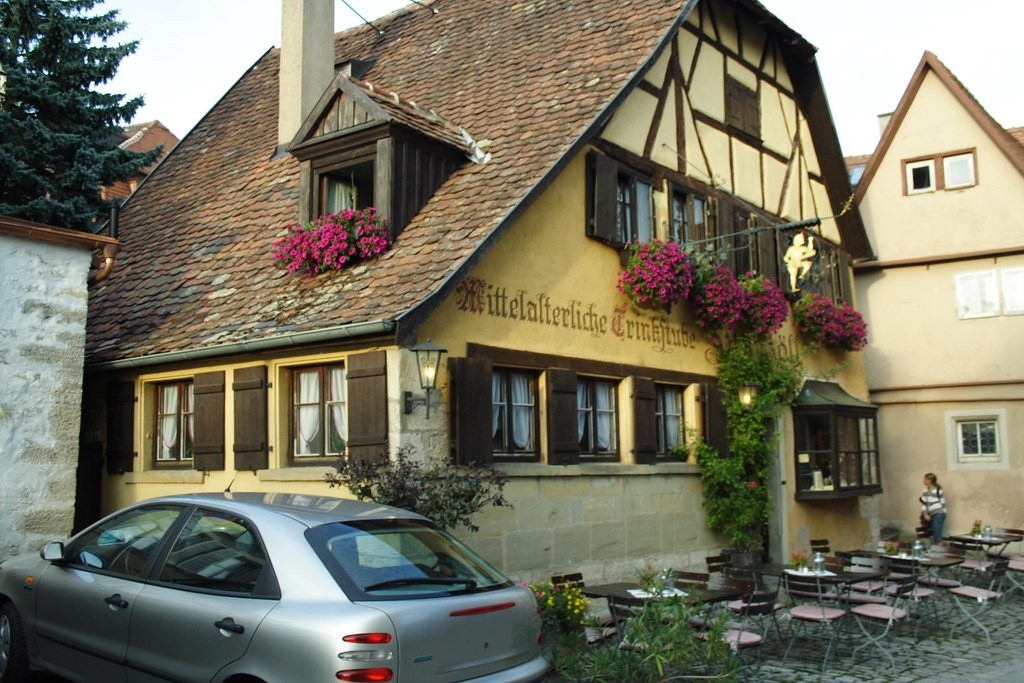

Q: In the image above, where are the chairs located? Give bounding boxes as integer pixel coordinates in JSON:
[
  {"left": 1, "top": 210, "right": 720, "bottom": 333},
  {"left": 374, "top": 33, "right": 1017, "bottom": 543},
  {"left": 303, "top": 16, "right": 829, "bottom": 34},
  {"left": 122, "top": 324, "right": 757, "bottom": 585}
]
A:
[{"left": 550, "top": 525, "right": 1024, "bottom": 678}]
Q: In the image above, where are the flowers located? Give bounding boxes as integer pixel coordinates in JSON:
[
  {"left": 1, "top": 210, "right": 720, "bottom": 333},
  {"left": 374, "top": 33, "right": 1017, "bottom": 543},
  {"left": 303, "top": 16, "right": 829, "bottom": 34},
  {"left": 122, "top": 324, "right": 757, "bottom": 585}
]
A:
[
  {"left": 792, "top": 292, "right": 840, "bottom": 349},
  {"left": 840, "top": 301, "right": 869, "bottom": 352},
  {"left": 617, "top": 236, "right": 693, "bottom": 314},
  {"left": 788, "top": 546, "right": 809, "bottom": 566},
  {"left": 742, "top": 271, "right": 789, "bottom": 335},
  {"left": 520, "top": 580, "right": 595, "bottom": 628},
  {"left": 272, "top": 204, "right": 394, "bottom": 276},
  {"left": 692, "top": 266, "right": 747, "bottom": 340}
]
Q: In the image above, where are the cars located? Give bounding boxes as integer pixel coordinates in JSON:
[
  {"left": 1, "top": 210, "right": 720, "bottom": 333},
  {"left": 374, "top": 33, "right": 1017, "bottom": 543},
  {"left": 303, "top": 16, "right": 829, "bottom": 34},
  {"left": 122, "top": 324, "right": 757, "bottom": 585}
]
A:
[{"left": 0, "top": 490, "right": 556, "bottom": 683}]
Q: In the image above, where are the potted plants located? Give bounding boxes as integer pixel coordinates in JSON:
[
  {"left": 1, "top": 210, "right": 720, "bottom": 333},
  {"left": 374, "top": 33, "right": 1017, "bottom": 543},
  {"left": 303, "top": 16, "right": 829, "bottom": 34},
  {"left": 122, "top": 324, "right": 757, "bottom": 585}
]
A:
[{"left": 672, "top": 337, "right": 847, "bottom": 566}]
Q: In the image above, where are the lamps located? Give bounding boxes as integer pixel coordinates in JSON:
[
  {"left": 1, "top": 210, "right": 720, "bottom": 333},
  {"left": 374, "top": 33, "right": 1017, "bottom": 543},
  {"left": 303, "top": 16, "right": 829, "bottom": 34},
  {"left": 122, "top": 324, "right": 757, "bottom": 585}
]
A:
[{"left": 403, "top": 339, "right": 448, "bottom": 419}]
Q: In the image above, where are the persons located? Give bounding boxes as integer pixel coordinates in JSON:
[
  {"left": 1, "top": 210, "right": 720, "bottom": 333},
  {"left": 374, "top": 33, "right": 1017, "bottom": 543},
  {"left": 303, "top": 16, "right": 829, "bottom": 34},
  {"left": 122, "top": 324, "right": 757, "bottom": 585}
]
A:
[{"left": 919, "top": 472, "right": 947, "bottom": 548}]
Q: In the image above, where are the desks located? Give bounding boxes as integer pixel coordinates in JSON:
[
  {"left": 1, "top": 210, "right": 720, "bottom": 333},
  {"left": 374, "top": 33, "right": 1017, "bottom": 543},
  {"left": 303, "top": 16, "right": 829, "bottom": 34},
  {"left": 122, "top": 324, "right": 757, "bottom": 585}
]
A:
[
  {"left": 735, "top": 565, "right": 883, "bottom": 656},
  {"left": 836, "top": 550, "right": 965, "bottom": 627},
  {"left": 949, "top": 534, "right": 1024, "bottom": 595},
  {"left": 579, "top": 581, "right": 737, "bottom": 649}
]
